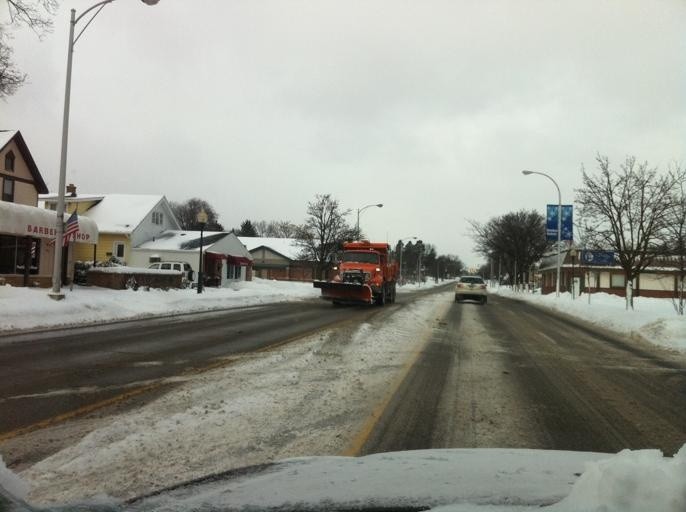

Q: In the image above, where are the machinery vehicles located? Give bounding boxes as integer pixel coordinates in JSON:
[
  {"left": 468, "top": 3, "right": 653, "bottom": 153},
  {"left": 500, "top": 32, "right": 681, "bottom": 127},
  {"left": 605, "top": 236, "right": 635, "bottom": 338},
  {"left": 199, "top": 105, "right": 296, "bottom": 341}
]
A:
[{"left": 312, "top": 242, "right": 401, "bottom": 305}]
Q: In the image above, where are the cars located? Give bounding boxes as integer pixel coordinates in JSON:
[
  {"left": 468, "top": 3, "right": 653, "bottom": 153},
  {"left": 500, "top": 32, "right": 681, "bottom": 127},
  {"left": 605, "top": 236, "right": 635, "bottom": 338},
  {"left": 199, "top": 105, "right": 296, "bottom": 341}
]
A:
[{"left": 454, "top": 276, "right": 488, "bottom": 302}]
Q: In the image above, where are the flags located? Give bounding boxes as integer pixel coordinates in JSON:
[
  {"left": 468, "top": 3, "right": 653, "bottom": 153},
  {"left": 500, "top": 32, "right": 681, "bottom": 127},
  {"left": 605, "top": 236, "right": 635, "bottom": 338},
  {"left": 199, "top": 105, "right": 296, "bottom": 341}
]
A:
[{"left": 63, "top": 210, "right": 79, "bottom": 247}]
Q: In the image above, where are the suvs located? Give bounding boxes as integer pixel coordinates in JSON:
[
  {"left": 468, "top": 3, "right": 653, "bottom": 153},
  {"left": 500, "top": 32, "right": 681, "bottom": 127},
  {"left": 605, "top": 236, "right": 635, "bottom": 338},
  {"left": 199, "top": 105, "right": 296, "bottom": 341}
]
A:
[{"left": 145, "top": 261, "right": 196, "bottom": 284}]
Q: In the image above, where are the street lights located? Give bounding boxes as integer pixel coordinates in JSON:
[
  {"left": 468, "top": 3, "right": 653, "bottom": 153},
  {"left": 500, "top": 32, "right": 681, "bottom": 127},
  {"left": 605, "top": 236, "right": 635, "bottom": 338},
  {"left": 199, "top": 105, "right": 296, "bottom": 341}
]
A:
[
  {"left": 197, "top": 207, "right": 208, "bottom": 293},
  {"left": 357, "top": 204, "right": 383, "bottom": 241},
  {"left": 47, "top": 0, "right": 159, "bottom": 302},
  {"left": 522, "top": 170, "right": 561, "bottom": 297}
]
